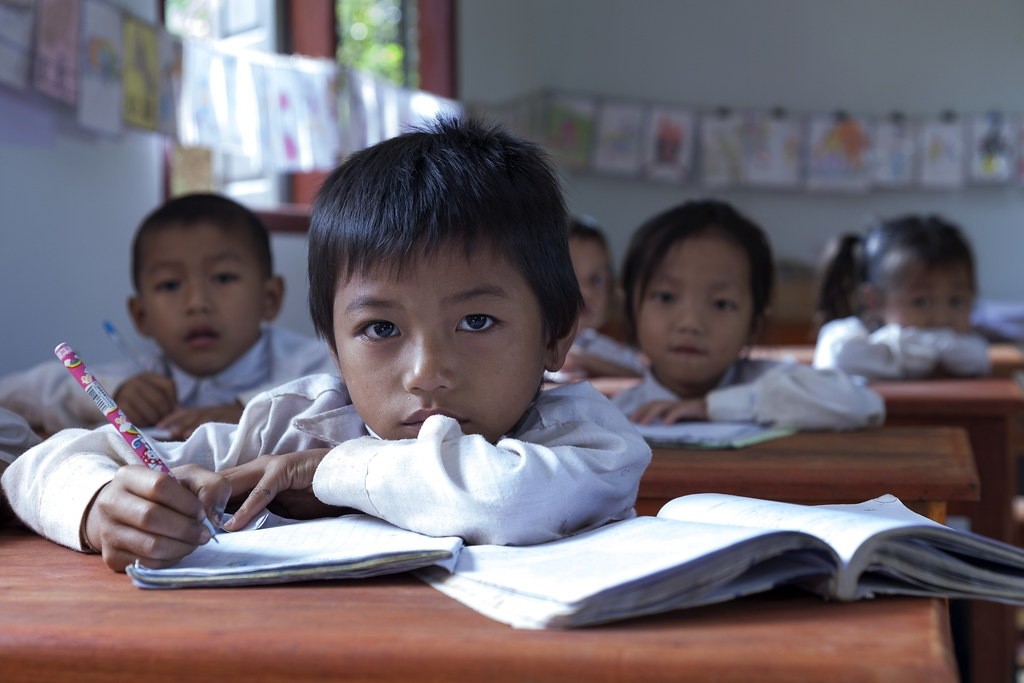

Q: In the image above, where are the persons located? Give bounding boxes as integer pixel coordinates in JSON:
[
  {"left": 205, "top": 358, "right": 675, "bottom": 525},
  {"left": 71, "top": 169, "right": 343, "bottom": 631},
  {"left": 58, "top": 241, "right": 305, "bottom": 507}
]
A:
[
  {"left": 543, "top": 219, "right": 650, "bottom": 384},
  {"left": 0, "top": 407, "right": 45, "bottom": 480},
  {"left": 813, "top": 214, "right": 1024, "bottom": 380},
  {"left": 0, "top": 191, "right": 338, "bottom": 439},
  {"left": 0, "top": 110, "right": 653, "bottom": 572},
  {"left": 611, "top": 200, "right": 885, "bottom": 431}
]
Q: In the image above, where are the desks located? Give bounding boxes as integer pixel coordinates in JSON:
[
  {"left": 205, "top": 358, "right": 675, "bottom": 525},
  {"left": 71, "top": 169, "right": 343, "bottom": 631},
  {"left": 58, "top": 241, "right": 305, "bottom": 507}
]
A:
[{"left": 0, "top": 344, "right": 1024, "bottom": 683}]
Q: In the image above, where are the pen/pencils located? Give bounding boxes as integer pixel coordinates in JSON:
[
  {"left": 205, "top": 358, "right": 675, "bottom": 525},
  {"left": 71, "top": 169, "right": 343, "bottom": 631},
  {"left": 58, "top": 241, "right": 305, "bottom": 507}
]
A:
[{"left": 54, "top": 342, "right": 219, "bottom": 545}]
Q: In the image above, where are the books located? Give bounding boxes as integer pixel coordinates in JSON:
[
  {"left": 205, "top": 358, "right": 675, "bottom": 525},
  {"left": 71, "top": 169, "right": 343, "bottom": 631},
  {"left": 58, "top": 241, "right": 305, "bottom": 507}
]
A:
[
  {"left": 628, "top": 423, "right": 793, "bottom": 448},
  {"left": 126, "top": 477, "right": 1024, "bottom": 634}
]
